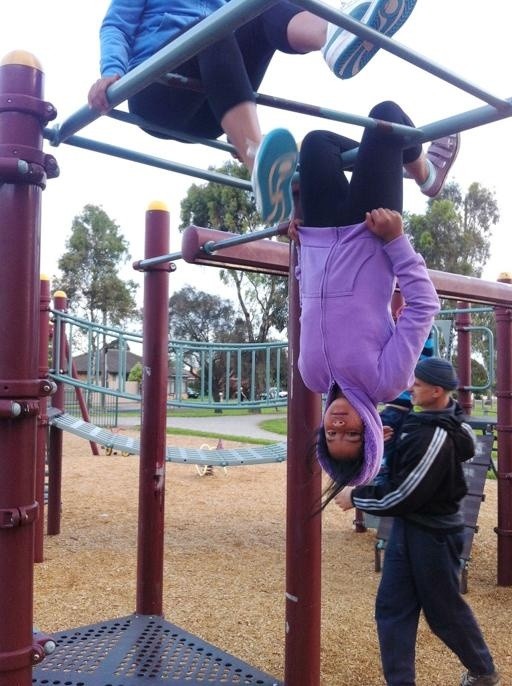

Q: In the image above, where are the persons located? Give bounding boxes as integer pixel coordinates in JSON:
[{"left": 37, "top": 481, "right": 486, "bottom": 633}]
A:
[
  {"left": 367, "top": 310, "right": 438, "bottom": 492},
  {"left": 284, "top": 99, "right": 462, "bottom": 489},
  {"left": 327, "top": 354, "right": 502, "bottom": 685},
  {"left": 85, "top": 0, "right": 422, "bottom": 228}
]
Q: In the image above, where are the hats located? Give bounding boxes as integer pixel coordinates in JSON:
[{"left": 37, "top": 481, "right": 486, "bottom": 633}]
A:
[{"left": 415, "top": 356, "right": 461, "bottom": 391}]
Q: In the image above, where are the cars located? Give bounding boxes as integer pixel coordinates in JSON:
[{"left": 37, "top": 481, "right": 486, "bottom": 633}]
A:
[
  {"left": 186, "top": 388, "right": 199, "bottom": 400},
  {"left": 260, "top": 386, "right": 287, "bottom": 399}
]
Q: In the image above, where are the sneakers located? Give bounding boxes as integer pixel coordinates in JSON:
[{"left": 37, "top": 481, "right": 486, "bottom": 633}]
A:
[
  {"left": 421, "top": 133, "right": 460, "bottom": 197},
  {"left": 321, "top": 1, "right": 417, "bottom": 81},
  {"left": 247, "top": 127, "right": 300, "bottom": 224},
  {"left": 460, "top": 671, "right": 503, "bottom": 686}
]
